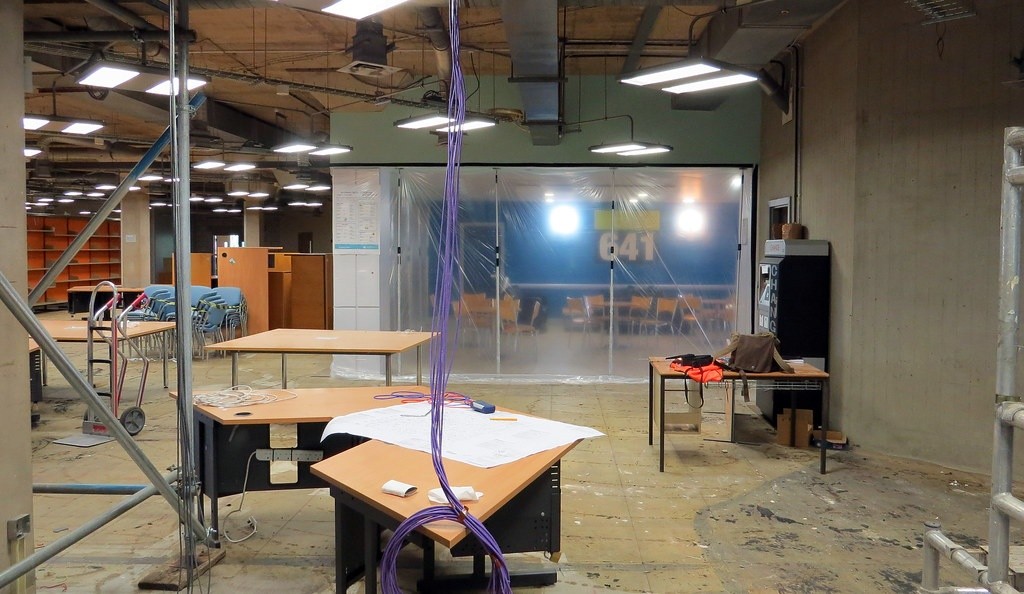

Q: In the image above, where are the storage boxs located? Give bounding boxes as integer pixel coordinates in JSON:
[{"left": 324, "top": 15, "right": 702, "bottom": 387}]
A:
[
  {"left": 813, "top": 430, "right": 847, "bottom": 449},
  {"left": 775, "top": 407, "right": 815, "bottom": 447}
]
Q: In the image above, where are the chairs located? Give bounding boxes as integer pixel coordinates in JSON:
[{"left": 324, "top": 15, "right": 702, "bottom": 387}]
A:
[
  {"left": 452, "top": 287, "right": 734, "bottom": 352},
  {"left": 124, "top": 283, "right": 249, "bottom": 364}
]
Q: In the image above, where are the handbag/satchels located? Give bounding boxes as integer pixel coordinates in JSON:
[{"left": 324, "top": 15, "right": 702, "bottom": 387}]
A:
[
  {"left": 715, "top": 331, "right": 796, "bottom": 374},
  {"left": 665, "top": 351, "right": 714, "bottom": 368}
]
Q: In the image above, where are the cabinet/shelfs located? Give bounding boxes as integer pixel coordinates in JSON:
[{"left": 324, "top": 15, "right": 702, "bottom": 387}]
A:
[{"left": 26, "top": 218, "right": 121, "bottom": 307}]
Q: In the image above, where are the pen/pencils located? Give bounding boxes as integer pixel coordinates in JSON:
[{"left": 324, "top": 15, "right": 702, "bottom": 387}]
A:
[{"left": 491, "top": 418, "right": 517, "bottom": 421}]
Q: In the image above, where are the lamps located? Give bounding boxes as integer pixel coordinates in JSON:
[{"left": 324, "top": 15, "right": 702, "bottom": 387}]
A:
[{"left": 23, "top": 0, "right": 788, "bottom": 213}]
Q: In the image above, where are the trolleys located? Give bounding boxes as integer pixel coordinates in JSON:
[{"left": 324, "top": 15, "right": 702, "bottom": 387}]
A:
[{"left": 53, "top": 281, "right": 150, "bottom": 448}]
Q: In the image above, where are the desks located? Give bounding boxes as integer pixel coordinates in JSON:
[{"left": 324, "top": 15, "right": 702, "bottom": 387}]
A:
[
  {"left": 470, "top": 299, "right": 502, "bottom": 346},
  {"left": 24, "top": 318, "right": 177, "bottom": 416},
  {"left": 648, "top": 358, "right": 828, "bottom": 472},
  {"left": 589, "top": 300, "right": 641, "bottom": 349},
  {"left": 171, "top": 244, "right": 335, "bottom": 335},
  {"left": 203, "top": 328, "right": 441, "bottom": 390},
  {"left": 29, "top": 338, "right": 58, "bottom": 423},
  {"left": 309, "top": 403, "right": 587, "bottom": 594},
  {"left": 167, "top": 385, "right": 470, "bottom": 545},
  {"left": 697, "top": 298, "right": 731, "bottom": 345}
]
